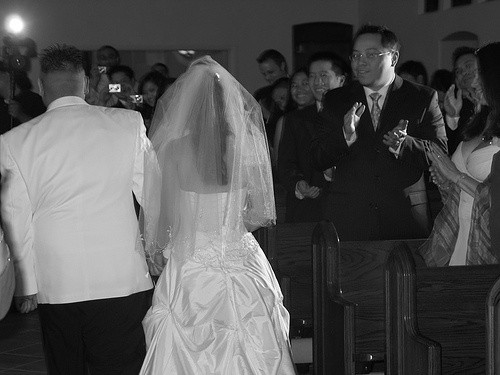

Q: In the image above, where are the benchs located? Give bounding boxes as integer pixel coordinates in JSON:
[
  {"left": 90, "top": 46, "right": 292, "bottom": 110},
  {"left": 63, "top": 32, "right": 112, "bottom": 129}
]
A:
[
  {"left": 257, "top": 186, "right": 380, "bottom": 375},
  {"left": 312, "top": 220, "right": 430, "bottom": 375},
  {"left": 386, "top": 241, "right": 500, "bottom": 375}
]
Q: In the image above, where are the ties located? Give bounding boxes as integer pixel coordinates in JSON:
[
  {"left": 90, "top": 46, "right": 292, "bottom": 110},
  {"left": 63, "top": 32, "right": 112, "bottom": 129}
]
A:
[{"left": 369, "top": 93, "right": 383, "bottom": 132}]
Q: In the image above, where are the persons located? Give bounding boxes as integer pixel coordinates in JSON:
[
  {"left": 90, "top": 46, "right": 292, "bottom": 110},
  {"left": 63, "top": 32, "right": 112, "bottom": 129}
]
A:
[
  {"left": 132, "top": 55, "right": 298, "bottom": 375},
  {"left": 85, "top": 45, "right": 177, "bottom": 137},
  {"left": 0, "top": 43, "right": 167, "bottom": 375},
  {"left": 250, "top": 24, "right": 500, "bottom": 269},
  {"left": 0, "top": 60, "right": 48, "bottom": 136}
]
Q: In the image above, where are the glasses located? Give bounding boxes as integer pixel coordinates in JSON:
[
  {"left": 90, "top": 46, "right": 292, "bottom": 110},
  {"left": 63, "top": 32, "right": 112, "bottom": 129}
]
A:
[{"left": 349, "top": 52, "right": 394, "bottom": 62}]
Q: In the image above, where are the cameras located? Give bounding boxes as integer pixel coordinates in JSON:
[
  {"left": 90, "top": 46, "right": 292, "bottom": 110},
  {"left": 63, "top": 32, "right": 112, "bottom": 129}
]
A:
[
  {"left": 129, "top": 95, "right": 144, "bottom": 103},
  {"left": 108, "top": 84, "right": 122, "bottom": 93},
  {"left": 97, "top": 65, "right": 106, "bottom": 75},
  {"left": 0, "top": 37, "right": 35, "bottom": 71}
]
